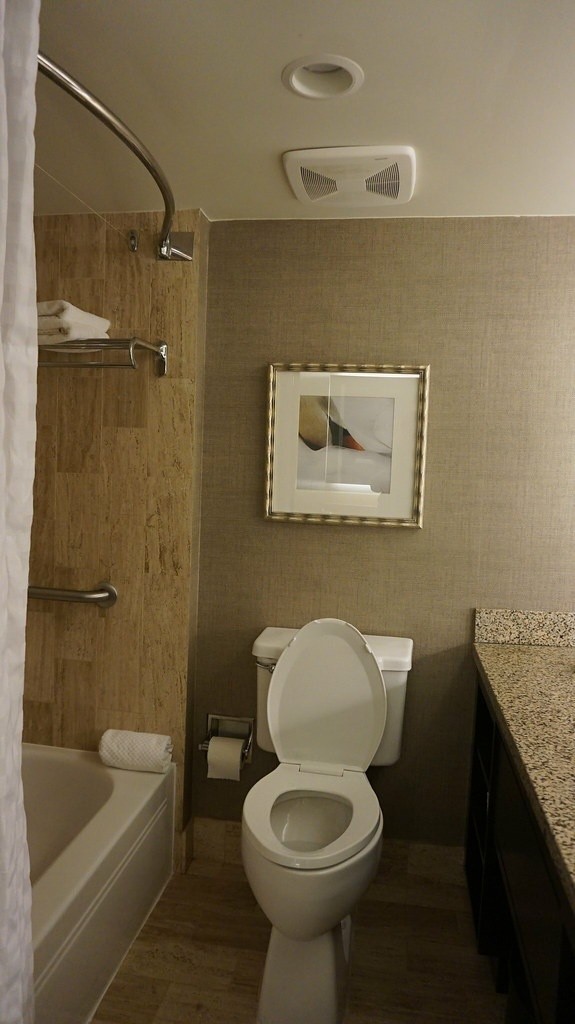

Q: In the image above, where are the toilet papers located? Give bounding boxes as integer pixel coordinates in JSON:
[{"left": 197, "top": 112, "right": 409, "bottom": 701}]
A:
[{"left": 206, "top": 736, "right": 246, "bottom": 782}]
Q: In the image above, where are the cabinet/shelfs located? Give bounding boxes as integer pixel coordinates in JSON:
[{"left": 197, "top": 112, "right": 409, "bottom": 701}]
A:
[{"left": 466, "top": 672, "right": 575, "bottom": 1024}]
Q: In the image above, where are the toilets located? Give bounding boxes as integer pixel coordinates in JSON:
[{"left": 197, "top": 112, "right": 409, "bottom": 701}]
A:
[{"left": 240, "top": 617, "right": 416, "bottom": 1024}]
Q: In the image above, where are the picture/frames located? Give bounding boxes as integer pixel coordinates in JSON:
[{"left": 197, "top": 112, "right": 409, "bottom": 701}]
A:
[{"left": 264, "top": 362, "right": 431, "bottom": 528}]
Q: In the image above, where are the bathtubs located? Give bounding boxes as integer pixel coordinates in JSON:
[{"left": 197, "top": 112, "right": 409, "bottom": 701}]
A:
[{"left": 20, "top": 739, "right": 175, "bottom": 1024}]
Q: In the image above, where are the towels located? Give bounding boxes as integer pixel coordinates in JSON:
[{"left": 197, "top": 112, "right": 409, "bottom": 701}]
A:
[
  {"left": 36, "top": 300, "right": 112, "bottom": 346},
  {"left": 99, "top": 728, "right": 173, "bottom": 774}
]
